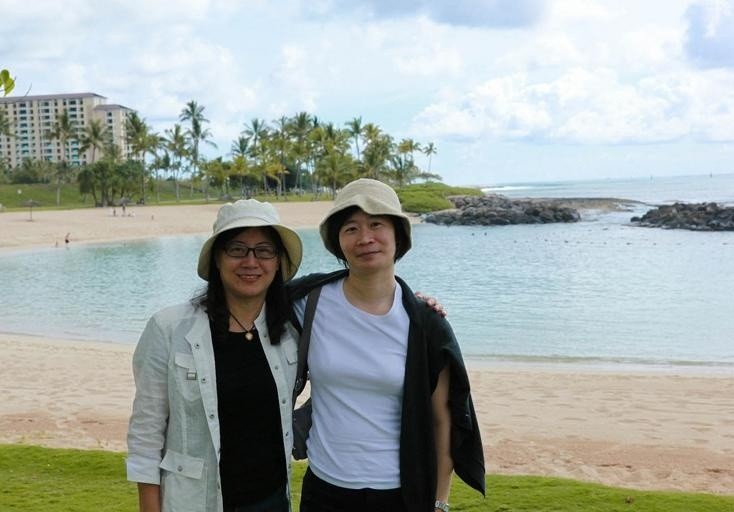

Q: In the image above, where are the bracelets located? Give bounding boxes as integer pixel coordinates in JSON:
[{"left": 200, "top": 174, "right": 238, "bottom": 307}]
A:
[{"left": 433, "top": 499, "right": 451, "bottom": 512}]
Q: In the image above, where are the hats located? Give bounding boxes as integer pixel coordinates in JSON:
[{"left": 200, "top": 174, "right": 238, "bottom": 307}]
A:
[
  {"left": 318, "top": 178, "right": 412, "bottom": 262},
  {"left": 197, "top": 198, "right": 303, "bottom": 283}
]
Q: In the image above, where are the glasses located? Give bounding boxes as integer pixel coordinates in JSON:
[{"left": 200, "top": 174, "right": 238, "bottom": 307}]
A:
[{"left": 220, "top": 242, "right": 278, "bottom": 259}]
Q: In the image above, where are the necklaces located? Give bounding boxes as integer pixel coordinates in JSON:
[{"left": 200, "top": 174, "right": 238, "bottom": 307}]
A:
[{"left": 230, "top": 312, "right": 257, "bottom": 342}]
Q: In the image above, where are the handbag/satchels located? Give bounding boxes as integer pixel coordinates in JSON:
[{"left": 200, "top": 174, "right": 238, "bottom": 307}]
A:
[{"left": 292, "top": 398, "right": 311, "bottom": 459}]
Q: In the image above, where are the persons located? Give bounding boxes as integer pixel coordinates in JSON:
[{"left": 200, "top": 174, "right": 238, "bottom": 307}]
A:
[
  {"left": 121, "top": 197, "right": 448, "bottom": 512},
  {"left": 286, "top": 176, "right": 487, "bottom": 512}
]
